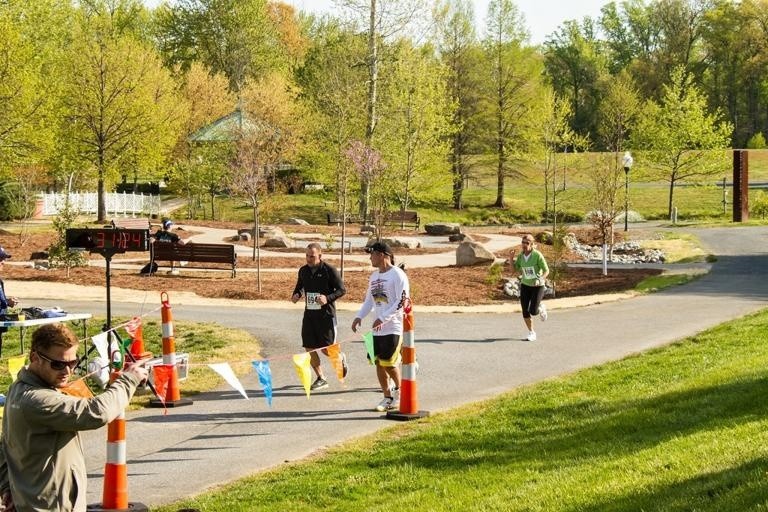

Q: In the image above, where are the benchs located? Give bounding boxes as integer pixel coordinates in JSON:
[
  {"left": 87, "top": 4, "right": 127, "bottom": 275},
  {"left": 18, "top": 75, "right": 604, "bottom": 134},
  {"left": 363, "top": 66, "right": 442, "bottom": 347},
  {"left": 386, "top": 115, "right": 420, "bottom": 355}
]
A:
[
  {"left": 145, "top": 240, "right": 237, "bottom": 282},
  {"left": 367, "top": 207, "right": 421, "bottom": 232}
]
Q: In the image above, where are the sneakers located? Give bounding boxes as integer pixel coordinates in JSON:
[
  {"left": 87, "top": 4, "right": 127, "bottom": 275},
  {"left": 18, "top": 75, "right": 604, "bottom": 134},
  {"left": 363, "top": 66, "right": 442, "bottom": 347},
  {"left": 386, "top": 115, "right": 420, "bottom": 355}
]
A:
[
  {"left": 539, "top": 303, "right": 547, "bottom": 322},
  {"left": 375, "top": 396, "right": 393, "bottom": 412},
  {"left": 170, "top": 269, "right": 180, "bottom": 275},
  {"left": 180, "top": 260, "right": 188, "bottom": 267},
  {"left": 310, "top": 376, "right": 329, "bottom": 391},
  {"left": 525, "top": 330, "right": 537, "bottom": 342},
  {"left": 388, "top": 387, "right": 401, "bottom": 409},
  {"left": 340, "top": 351, "right": 350, "bottom": 380}
]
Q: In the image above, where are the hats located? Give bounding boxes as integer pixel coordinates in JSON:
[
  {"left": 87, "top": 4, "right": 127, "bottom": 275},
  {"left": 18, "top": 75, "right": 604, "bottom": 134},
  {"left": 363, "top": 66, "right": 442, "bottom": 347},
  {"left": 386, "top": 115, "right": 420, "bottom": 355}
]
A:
[
  {"left": 163, "top": 220, "right": 175, "bottom": 227},
  {"left": 364, "top": 241, "right": 393, "bottom": 257},
  {"left": 0, "top": 245, "right": 12, "bottom": 259}
]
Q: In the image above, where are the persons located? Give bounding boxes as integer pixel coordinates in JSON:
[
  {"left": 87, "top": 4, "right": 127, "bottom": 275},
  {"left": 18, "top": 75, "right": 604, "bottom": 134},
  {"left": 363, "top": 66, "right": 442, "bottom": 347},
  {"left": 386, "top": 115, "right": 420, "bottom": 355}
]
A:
[
  {"left": 290, "top": 243, "right": 347, "bottom": 392},
  {"left": 509, "top": 235, "right": 550, "bottom": 342},
  {"left": 0, "top": 321, "right": 156, "bottom": 512},
  {"left": 350, "top": 241, "right": 410, "bottom": 413},
  {"left": 149, "top": 218, "right": 194, "bottom": 276},
  {"left": 1, "top": 246, "right": 20, "bottom": 360}
]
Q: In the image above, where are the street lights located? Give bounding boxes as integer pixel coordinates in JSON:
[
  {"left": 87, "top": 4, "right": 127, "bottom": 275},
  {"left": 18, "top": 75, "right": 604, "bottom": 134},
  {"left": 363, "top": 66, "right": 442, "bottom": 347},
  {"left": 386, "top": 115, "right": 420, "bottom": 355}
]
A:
[{"left": 622, "top": 150, "right": 634, "bottom": 232}]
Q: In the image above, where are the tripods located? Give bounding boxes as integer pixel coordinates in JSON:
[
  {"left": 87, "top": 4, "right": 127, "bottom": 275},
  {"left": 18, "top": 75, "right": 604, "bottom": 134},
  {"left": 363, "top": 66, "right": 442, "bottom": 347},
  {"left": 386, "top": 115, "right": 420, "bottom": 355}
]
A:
[{"left": 74, "top": 264, "right": 162, "bottom": 400}]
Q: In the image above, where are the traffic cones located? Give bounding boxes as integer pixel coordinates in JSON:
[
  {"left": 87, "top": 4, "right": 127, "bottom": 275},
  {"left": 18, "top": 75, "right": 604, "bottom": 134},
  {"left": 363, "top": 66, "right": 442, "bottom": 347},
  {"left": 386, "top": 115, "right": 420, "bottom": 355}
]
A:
[
  {"left": 384, "top": 294, "right": 432, "bottom": 421},
  {"left": 86, "top": 348, "right": 149, "bottom": 512},
  {"left": 145, "top": 292, "right": 195, "bottom": 409}
]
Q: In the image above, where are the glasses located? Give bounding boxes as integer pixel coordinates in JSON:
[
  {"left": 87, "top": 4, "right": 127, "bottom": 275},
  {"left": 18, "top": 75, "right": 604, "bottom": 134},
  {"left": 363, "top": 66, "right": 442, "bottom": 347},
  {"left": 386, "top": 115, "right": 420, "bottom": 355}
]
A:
[
  {"left": 32, "top": 348, "right": 80, "bottom": 371},
  {"left": 521, "top": 241, "right": 531, "bottom": 245}
]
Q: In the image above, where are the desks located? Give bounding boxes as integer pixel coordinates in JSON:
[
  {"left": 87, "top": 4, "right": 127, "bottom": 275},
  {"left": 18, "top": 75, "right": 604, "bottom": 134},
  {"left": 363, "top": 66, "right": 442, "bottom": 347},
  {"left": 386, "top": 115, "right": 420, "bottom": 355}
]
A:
[{"left": 0, "top": 311, "right": 93, "bottom": 386}]
